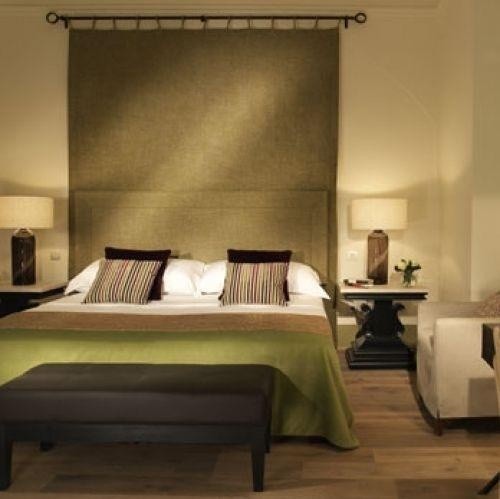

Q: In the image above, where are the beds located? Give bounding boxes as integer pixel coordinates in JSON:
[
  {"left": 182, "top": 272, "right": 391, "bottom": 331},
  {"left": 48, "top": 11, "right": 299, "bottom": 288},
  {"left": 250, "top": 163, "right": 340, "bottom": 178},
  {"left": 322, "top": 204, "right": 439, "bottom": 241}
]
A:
[{"left": 0, "top": 191, "right": 336, "bottom": 450}]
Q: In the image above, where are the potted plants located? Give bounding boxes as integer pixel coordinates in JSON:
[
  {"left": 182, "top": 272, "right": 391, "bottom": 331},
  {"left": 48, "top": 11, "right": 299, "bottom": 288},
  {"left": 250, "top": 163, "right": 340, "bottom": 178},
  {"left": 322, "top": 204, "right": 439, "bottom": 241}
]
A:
[{"left": 394, "top": 258, "right": 420, "bottom": 284}]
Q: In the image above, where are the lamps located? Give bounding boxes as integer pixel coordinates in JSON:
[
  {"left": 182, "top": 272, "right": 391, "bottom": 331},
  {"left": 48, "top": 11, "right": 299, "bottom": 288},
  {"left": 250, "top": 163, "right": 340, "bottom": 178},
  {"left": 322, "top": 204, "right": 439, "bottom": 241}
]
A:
[
  {"left": 352, "top": 198, "right": 407, "bottom": 284},
  {"left": 0, "top": 197, "right": 54, "bottom": 285}
]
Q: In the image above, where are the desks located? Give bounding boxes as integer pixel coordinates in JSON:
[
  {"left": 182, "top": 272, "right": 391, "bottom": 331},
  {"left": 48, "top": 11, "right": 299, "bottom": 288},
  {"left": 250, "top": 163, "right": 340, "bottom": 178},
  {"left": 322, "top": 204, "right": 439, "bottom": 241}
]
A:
[
  {"left": 339, "top": 283, "right": 428, "bottom": 370},
  {"left": 0, "top": 283, "right": 63, "bottom": 310},
  {"left": 476, "top": 323, "right": 500, "bottom": 494}
]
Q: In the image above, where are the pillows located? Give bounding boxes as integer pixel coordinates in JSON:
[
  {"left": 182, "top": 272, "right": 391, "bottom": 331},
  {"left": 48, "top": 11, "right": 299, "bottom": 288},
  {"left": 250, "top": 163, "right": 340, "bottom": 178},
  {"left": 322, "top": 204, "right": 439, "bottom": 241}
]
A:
[
  {"left": 469, "top": 290, "right": 500, "bottom": 317},
  {"left": 65, "top": 245, "right": 329, "bottom": 304}
]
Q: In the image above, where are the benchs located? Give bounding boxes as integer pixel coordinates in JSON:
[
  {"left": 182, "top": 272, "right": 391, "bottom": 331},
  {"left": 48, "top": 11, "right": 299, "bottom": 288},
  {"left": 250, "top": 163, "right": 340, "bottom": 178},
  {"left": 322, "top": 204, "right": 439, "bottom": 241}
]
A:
[{"left": 0, "top": 362, "right": 274, "bottom": 492}]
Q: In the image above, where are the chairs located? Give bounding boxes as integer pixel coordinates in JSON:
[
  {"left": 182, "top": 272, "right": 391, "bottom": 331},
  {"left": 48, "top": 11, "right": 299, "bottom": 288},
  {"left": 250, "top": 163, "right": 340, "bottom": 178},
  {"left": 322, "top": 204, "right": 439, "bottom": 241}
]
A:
[{"left": 416, "top": 302, "right": 500, "bottom": 439}]
